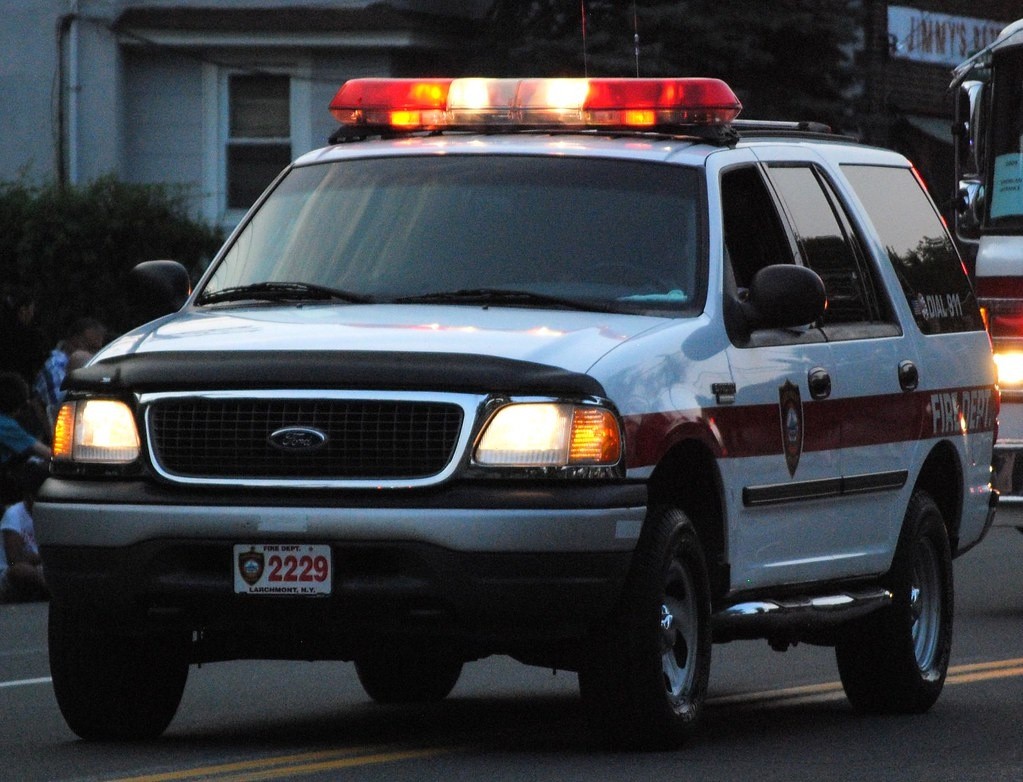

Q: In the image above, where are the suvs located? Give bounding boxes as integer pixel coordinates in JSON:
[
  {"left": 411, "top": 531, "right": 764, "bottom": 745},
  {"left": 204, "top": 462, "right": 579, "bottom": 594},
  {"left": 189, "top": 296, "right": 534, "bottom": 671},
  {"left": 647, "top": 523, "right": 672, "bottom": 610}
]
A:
[{"left": 29, "top": 77, "right": 1003, "bottom": 750}]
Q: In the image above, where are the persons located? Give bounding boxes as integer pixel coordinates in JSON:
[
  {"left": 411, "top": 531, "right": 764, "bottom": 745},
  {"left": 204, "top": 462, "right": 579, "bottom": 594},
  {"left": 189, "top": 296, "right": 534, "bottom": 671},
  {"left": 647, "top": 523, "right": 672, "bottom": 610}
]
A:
[
  {"left": 0, "top": 273, "right": 54, "bottom": 447},
  {"left": 44, "top": 317, "right": 106, "bottom": 429},
  {"left": 67, "top": 350, "right": 93, "bottom": 375},
  {"left": 0, "top": 477, "right": 52, "bottom": 605},
  {"left": 1, "top": 374, "right": 52, "bottom": 520}
]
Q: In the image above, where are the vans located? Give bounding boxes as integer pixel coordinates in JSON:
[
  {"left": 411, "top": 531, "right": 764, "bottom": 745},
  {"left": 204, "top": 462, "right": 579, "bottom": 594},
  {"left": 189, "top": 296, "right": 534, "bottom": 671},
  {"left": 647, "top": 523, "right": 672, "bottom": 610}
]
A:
[{"left": 945, "top": 16, "right": 1023, "bottom": 508}]
